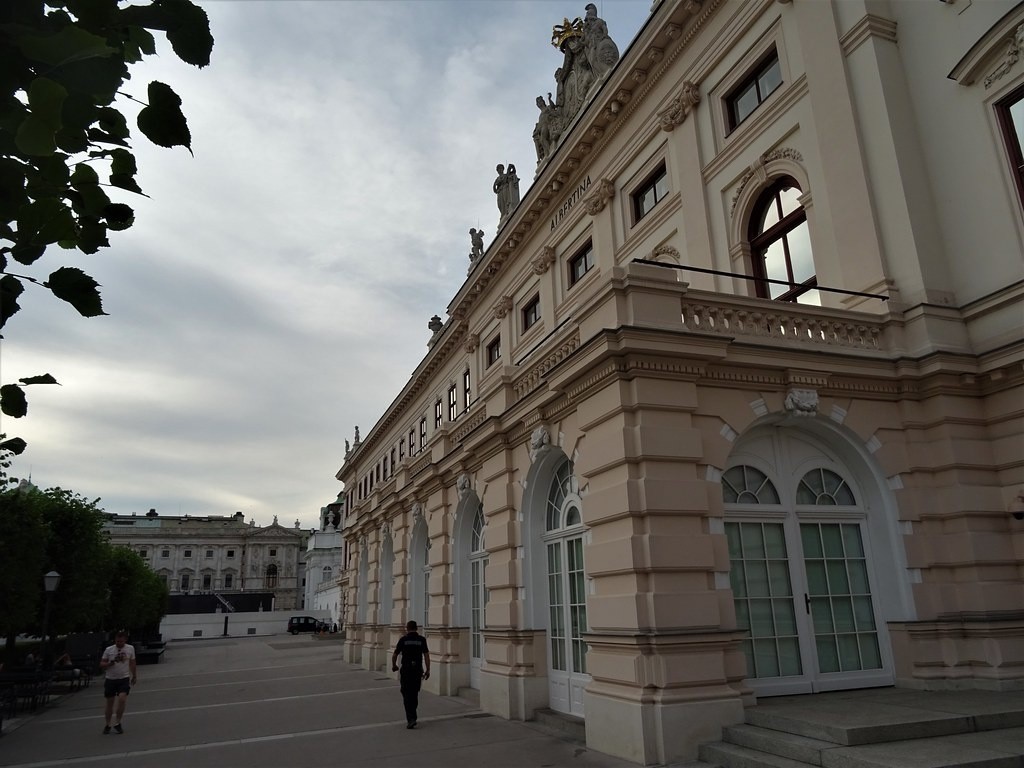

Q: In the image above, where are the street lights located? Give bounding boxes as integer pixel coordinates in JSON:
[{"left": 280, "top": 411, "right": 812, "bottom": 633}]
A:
[{"left": 29, "top": 569, "right": 63, "bottom": 712}]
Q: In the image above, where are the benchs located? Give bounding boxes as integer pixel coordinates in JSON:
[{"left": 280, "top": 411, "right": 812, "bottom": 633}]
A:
[{"left": 0, "top": 656, "right": 96, "bottom": 736}]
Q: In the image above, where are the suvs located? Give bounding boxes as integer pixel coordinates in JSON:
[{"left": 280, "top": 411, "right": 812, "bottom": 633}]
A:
[{"left": 286, "top": 615, "right": 328, "bottom": 635}]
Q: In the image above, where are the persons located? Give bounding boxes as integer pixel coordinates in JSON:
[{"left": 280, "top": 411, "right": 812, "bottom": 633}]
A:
[
  {"left": 493, "top": 163, "right": 516, "bottom": 217},
  {"left": 392, "top": 619, "right": 431, "bottom": 729},
  {"left": 532, "top": 91, "right": 554, "bottom": 157},
  {"left": 354, "top": 426, "right": 360, "bottom": 441},
  {"left": 469, "top": 228, "right": 486, "bottom": 258},
  {"left": 577, "top": 4, "right": 609, "bottom": 68},
  {"left": 71, "top": 632, "right": 136, "bottom": 734}
]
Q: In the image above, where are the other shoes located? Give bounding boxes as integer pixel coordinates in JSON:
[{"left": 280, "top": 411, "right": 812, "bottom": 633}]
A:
[
  {"left": 407, "top": 719, "right": 416, "bottom": 729},
  {"left": 104, "top": 725, "right": 111, "bottom": 733},
  {"left": 113, "top": 723, "right": 123, "bottom": 733}
]
